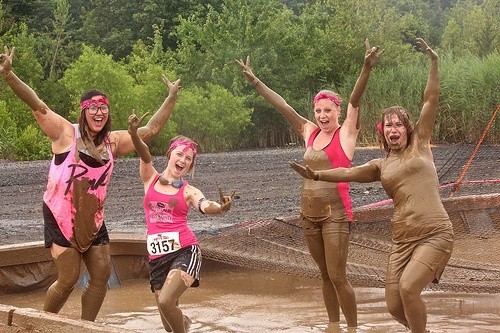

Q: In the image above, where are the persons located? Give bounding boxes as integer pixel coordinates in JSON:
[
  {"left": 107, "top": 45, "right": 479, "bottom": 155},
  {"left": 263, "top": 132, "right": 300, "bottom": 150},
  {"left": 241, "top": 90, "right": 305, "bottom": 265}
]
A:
[
  {"left": 0, "top": 45, "right": 182, "bottom": 321},
  {"left": 127, "top": 109, "right": 236, "bottom": 333},
  {"left": 235, "top": 38, "right": 386, "bottom": 327},
  {"left": 288, "top": 38, "right": 454, "bottom": 333}
]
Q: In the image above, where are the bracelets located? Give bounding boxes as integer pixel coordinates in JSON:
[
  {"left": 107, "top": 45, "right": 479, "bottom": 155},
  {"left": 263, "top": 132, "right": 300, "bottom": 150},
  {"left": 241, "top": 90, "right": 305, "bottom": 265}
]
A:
[
  {"left": 313, "top": 171, "right": 321, "bottom": 181},
  {"left": 131, "top": 133, "right": 139, "bottom": 140},
  {"left": 4, "top": 69, "right": 14, "bottom": 79},
  {"left": 250, "top": 76, "right": 259, "bottom": 85}
]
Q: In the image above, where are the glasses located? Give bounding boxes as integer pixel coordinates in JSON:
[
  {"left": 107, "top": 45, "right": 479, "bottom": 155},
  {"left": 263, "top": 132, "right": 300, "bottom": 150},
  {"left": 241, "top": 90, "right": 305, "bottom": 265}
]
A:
[
  {"left": 85, "top": 104, "right": 109, "bottom": 115},
  {"left": 159, "top": 170, "right": 183, "bottom": 188}
]
